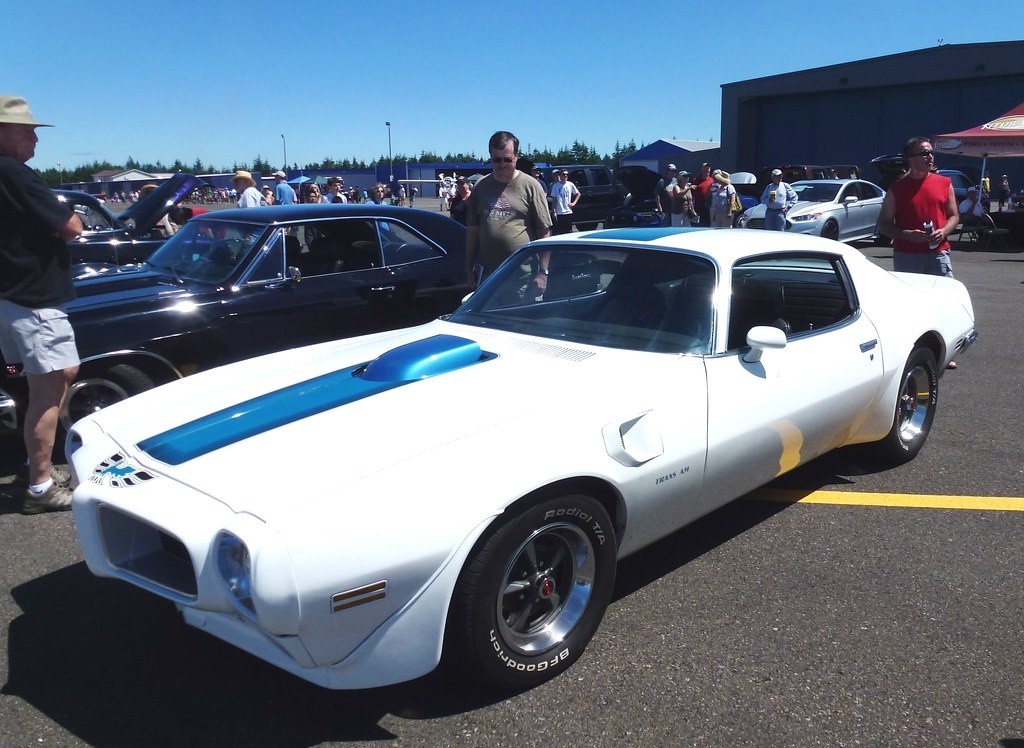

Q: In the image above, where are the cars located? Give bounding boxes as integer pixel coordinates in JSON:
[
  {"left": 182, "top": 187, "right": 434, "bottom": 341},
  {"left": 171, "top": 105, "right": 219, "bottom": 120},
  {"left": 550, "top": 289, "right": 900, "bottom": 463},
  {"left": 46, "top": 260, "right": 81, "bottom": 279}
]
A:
[
  {"left": 48, "top": 172, "right": 210, "bottom": 267},
  {"left": 535, "top": 165, "right": 621, "bottom": 232},
  {"left": 758, "top": 166, "right": 841, "bottom": 197},
  {"left": 735, "top": 178, "right": 888, "bottom": 245},
  {"left": 869, "top": 151, "right": 990, "bottom": 239},
  {"left": 2, "top": 204, "right": 605, "bottom": 457},
  {"left": 61, "top": 226, "right": 979, "bottom": 697}
]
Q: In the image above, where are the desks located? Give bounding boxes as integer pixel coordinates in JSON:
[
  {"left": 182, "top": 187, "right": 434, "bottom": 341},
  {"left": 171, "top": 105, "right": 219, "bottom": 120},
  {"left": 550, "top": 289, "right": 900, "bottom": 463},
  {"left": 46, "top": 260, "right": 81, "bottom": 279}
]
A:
[{"left": 988, "top": 209, "right": 1024, "bottom": 252}]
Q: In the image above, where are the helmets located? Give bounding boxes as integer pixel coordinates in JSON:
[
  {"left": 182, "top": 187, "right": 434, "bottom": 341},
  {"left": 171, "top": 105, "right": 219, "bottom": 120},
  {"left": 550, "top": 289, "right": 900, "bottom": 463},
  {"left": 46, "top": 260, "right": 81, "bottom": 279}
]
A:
[{"left": 772, "top": 169, "right": 782, "bottom": 177}]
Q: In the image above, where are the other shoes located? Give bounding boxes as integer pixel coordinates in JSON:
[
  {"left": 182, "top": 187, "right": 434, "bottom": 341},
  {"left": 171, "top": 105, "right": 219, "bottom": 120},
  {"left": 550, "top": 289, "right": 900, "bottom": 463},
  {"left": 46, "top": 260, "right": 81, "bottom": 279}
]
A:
[{"left": 948, "top": 360, "right": 957, "bottom": 368}]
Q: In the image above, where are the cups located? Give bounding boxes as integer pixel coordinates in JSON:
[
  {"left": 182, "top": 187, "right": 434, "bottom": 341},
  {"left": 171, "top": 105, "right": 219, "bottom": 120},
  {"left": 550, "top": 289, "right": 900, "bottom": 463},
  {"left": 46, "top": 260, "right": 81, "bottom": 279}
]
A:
[
  {"left": 770, "top": 191, "right": 776, "bottom": 200},
  {"left": 924, "top": 221, "right": 940, "bottom": 249}
]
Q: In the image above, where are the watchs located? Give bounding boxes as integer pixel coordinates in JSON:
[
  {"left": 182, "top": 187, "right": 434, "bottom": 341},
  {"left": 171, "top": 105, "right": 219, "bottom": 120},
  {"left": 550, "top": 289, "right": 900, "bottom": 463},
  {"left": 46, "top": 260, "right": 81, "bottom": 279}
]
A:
[{"left": 540, "top": 265, "right": 550, "bottom": 276}]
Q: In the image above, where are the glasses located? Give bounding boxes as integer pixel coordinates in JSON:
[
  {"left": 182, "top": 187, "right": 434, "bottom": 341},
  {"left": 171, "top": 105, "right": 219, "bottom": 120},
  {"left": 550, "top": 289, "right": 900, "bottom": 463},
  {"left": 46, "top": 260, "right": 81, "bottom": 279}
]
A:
[
  {"left": 374, "top": 190, "right": 384, "bottom": 193},
  {"left": 562, "top": 174, "right": 570, "bottom": 176},
  {"left": 309, "top": 196, "right": 317, "bottom": 198},
  {"left": 910, "top": 150, "right": 936, "bottom": 157},
  {"left": 490, "top": 155, "right": 516, "bottom": 163}
]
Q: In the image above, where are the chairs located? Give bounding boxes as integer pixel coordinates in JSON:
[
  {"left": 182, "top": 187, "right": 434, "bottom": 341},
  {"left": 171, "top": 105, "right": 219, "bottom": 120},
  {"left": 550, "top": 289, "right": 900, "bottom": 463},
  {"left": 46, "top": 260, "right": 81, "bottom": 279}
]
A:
[
  {"left": 271, "top": 234, "right": 433, "bottom": 276},
  {"left": 959, "top": 211, "right": 1011, "bottom": 251},
  {"left": 602, "top": 277, "right": 846, "bottom": 336}
]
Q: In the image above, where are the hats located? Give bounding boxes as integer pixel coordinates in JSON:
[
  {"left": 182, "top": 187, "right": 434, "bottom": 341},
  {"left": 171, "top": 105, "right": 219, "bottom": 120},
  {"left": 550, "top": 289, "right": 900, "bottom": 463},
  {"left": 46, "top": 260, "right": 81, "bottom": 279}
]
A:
[
  {"left": 349, "top": 187, "right": 352, "bottom": 189},
  {"left": 531, "top": 166, "right": 542, "bottom": 170},
  {"left": 966, "top": 187, "right": 978, "bottom": 192},
  {"left": 700, "top": 162, "right": 713, "bottom": 167},
  {"left": 666, "top": 164, "right": 678, "bottom": 171},
  {"left": 263, "top": 185, "right": 269, "bottom": 188},
  {"left": 231, "top": 171, "right": 256, "bottom": 186},
  {"left": 675, "top": 171, "right": 692, "bottom": 178},
  {"left": 552, "top": 169, "right": 562, "bottom": 174},
  {"left": 272, "top": 171, "right": 286, "bottom": 178},
  {"left": 0, "top": 96, "right": 55, "bottom": 127},
  {"left": 455, "top": 176, "right": 470, "bottom": 183},
  {"left": 712, "top": 169, "right": 730, "bottom": 185},
  {"left": 1002, "top": 175, "right": 1008, "bottom": 178}
]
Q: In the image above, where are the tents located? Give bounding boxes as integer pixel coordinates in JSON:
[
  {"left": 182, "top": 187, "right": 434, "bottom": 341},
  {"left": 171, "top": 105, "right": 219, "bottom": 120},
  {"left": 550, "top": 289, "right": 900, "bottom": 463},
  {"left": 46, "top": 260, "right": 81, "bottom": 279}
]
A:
[{"left": 932, "top": 103, "right": 1024, "bottom": 202}]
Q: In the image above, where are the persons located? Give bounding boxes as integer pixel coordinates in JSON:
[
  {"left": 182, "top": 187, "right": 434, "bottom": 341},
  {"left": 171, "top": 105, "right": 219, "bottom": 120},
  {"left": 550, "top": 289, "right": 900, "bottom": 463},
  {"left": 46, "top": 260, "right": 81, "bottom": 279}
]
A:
[
  {"left": 878, "top": 137, "right": 961, "bottom": 368},
  {"left": 657, "top": 164, "right": 859, "bottom": 232},
  {"left": 101, "top": 132, "right": 582, "bottom": 312},
  {"left": 958, "top": 170, "right": 1010, "bottom": 225},
  {"left": 0, "top": 92, "right": 84, "bottom": 514}
]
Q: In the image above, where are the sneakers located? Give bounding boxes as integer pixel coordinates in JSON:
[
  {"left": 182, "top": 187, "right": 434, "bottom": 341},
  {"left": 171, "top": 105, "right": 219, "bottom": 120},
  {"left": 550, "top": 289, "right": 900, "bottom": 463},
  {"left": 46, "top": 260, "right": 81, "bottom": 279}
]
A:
[{"left": 21, "top": 461, "right": 74, "bottom": 514}]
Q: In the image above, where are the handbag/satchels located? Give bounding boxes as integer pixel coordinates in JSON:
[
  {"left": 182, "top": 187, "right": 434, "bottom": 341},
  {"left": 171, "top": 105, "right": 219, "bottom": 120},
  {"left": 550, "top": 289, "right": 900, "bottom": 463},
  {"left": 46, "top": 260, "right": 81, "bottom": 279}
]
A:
[
  {"left": 450, "top": 195, "right": 457, "bottom": 200},
  {"left": 688, "top": 205, "right": 697, "bottom": 217},
  {"left": 726, "top": 185, "right": 742, "bottom": 212}
]
